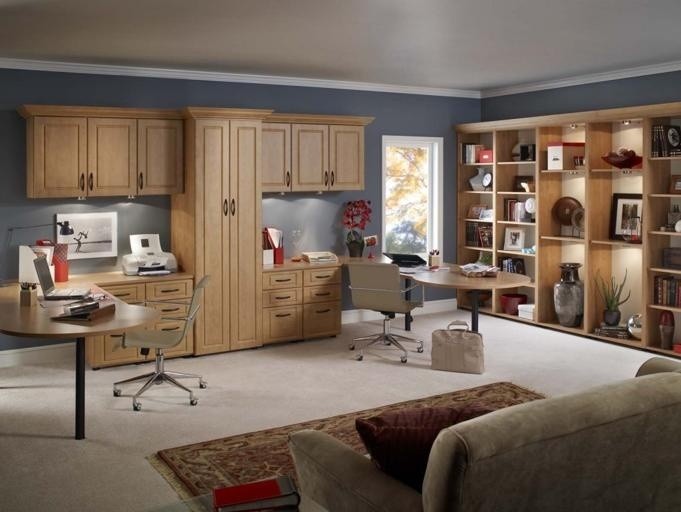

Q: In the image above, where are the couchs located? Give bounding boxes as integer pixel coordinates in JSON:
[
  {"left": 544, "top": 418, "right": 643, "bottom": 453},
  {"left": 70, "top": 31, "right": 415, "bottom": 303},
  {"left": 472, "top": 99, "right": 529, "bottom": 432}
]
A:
[{"left": 287, "top": 356, "right": 681, "bottom": 512}]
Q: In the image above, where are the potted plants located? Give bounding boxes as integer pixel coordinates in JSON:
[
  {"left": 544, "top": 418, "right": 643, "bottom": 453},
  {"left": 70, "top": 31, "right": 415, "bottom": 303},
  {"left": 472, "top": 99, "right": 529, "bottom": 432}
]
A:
[{"left": 592, "top": 267, "right": 633, "bottom": 326}]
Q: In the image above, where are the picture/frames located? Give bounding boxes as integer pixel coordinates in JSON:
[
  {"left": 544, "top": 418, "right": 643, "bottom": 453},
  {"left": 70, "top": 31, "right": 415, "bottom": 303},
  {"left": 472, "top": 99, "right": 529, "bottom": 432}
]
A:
[
  {"left": 56, "top": 212, "right": 118, "bottom": 261},
  {"left": 609, "top": 193, "right": 644, "bottom": 240},
  {"left": 504, "top": 226, "right": 526, "bottom": 251}
]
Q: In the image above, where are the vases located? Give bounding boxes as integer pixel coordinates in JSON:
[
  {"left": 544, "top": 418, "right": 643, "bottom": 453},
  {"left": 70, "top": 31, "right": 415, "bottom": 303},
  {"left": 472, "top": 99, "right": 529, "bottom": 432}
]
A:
[
  {"left": 552, "top": 263, "right": 585, "bottom": 327},
  {"left": 346, "top": 243, "right": 365, "bottom": 257}
]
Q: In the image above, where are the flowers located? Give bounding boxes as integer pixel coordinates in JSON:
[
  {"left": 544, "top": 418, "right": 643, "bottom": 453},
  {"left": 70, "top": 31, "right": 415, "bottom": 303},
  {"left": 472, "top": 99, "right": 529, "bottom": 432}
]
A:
[{"left": 339, "top": 199, "right": 373, "bottom": 242}]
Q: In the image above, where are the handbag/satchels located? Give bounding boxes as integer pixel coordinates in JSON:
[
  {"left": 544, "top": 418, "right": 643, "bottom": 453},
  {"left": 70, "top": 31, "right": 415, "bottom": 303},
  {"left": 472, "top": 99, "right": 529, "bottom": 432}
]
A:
[{"left": 431, "top": 321, "right": 485, "bottom": 374}]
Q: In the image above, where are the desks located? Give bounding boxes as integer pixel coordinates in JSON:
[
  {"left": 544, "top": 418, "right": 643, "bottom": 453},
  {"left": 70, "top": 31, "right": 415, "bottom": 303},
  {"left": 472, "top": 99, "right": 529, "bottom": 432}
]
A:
[
  {"left": 1, "top": 280, "right": 165, "bottom": 442},
  {"left": 126, "top": 479, "right": 336, "bottom": 512},
  {"left": 340, "top": 254, "right": 534, "bottom": 334}
]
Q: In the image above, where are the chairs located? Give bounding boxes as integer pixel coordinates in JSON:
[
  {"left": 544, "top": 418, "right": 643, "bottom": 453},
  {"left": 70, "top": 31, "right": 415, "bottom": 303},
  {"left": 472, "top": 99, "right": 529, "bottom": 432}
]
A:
[
  {"left": 348, "top": 261, "right": 427, "bottom": 365},
  {"left": 113, "top": 273, "right": 214, "bottom": 413}
]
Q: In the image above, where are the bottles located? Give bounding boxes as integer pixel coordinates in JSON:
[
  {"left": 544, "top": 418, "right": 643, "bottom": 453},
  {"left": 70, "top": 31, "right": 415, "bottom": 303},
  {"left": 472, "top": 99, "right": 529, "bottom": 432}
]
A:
[{"left": 624, "top": 216, "right": 642, "bottom": 242}]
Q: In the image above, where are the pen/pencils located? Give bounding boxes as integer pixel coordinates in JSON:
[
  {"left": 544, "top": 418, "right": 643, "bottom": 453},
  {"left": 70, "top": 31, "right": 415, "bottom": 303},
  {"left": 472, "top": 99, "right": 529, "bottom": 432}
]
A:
[
  {"left": 20, "top": 282, "right": 37, "bottom": 290},
  {"left": 430, "top": 249, "right": 439, "bottom": 255},
  {"left": 39, "top": 303, "right": 45, "bottom": 308}
]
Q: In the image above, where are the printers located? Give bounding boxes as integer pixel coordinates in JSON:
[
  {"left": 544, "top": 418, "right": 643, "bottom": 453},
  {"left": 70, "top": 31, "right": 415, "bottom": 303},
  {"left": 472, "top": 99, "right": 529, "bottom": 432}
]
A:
[{"left": 122, "top": 234, "right": 177, "bottom": 276}]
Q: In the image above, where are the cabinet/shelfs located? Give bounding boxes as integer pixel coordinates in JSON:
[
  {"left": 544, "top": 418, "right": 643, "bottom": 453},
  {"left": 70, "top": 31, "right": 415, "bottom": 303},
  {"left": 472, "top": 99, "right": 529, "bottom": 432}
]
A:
[
  {"left": 645, "top": 111, "right": 681, "bottom": 358},
  {"left": 171, "top": 119, "right": 262, "bottom": 356},
  {"left": 138, "top": 119, "right": 186, "bottom": 196},
  {"left": 293, "top": 122, "right": 366, "bottom": 193},
  {"left": 588, "top": 115, "right": 645, "bottom": 350},
  {"left": 262, "top": 266, "right": 344, "bottom": 345},
  {"left": 82, "top": 279, "right": 195, "bottom": 372},
  {"left": 261, "top": 122, "right": 293, "bottom": 195},
  {"left": 26, "top": 116, "right": 137, "bottom": 200},
  {"left": 539, "top": 120, "right": 588, "bottom": 337},
  {"left": 495, "top": 125, "right": 539, "bottom": 325},
  {"left": 457, "top": 127, "right": 495, "bottom": 316}
]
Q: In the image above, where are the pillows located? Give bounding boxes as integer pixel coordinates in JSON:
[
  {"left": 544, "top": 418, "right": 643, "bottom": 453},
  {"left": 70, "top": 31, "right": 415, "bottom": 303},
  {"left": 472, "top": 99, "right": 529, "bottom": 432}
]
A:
[{"left": 354, "top": 405, "right": 498, "bottom": 495}]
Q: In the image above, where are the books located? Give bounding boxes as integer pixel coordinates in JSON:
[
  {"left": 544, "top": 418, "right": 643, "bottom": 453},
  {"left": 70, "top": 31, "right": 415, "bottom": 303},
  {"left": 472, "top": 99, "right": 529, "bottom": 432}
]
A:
[
  {"left": 455, "top": 262, "right": 499, "bottom": 278},
  {"left": 463, "top": 143, "right": 485, "bottom": 163},
  {"left": 652, "top": 125, "right": 681, "bottom": 157},
  {"left": 519, "top": 304, "right": 535, "bottom": 320},
  {"left": 654, "top": 275, "right": 681, "bottom": 308},
  {"left": 63, "top": 297, "right": 100, "bottom": 314},
  {"left": 303, "top": 251, "right": 338, "bottom": 263},
  {"left": 504, "top": 198, "right": 526, "bottom": 222},
  {"left": 466, "top": 222, "right": 493, "bottom": 247},
  {"left": 267, "top": 227, "right": 283, "bottom": 248},
  {"left": 594, "top": 320, "right": 631, "bottom": 339},
  {"left": 498, "top": 256, "right": 526, "bottom": 275}
]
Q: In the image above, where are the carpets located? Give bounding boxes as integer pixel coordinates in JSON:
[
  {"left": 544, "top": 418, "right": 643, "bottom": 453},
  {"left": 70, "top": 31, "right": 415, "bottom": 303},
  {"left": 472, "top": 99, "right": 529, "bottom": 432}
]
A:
[{"left": 140, "top": 380, "right": 548, "bottom": 512}]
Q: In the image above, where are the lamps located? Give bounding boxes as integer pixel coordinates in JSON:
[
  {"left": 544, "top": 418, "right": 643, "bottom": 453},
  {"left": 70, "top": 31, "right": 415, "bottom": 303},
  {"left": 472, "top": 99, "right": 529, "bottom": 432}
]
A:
[{"left": 7, "top": 219, "right": 75, "bottom": 249}]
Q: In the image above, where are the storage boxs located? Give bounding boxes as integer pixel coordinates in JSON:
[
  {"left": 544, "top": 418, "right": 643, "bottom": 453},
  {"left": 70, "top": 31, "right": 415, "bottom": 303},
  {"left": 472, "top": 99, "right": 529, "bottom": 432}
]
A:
[{"left": 547, "top": 141, "right": 586, "bottom": 171}]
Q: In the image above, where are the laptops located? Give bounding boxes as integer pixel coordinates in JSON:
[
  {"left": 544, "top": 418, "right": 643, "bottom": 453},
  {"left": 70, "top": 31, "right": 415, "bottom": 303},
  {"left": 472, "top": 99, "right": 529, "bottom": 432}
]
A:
[
  {"left": 33, "top": 256, "right": 90, "bottom": 300},
  {"left": 382, "top": 251, "right": 426, "bottom": 273}
]
